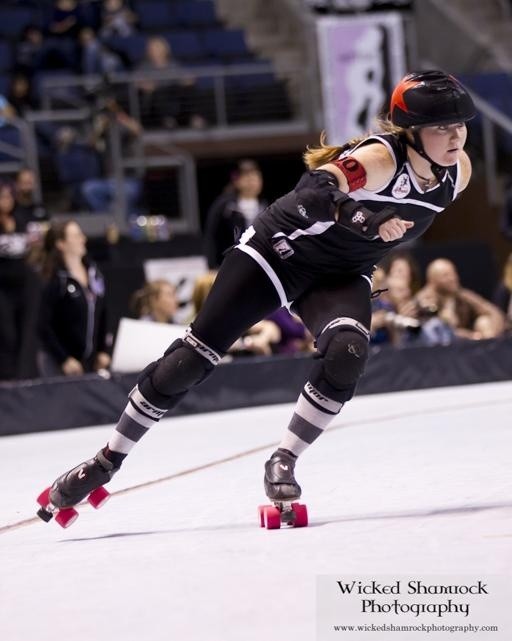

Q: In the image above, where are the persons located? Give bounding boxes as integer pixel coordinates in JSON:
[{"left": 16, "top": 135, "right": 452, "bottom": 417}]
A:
[
  {"left": 2, "top": 1, "right": 511, "bottom": 373},
  {"left": 49, "top": 72, "right": 479, "bottom": 518}
]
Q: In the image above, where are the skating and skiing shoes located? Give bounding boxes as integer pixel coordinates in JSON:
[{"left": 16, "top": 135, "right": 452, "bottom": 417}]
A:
[
  {"left": 35, "top": 450, "right": 118, "bottom": 526},
  {"left": 259, "top": 450, "right": 308, "bottom": 529}
]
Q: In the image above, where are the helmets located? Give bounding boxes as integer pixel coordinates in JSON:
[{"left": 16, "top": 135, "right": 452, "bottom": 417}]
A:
[{"left": 390, "top": 68, "right": 476, "bottom": 128}]
